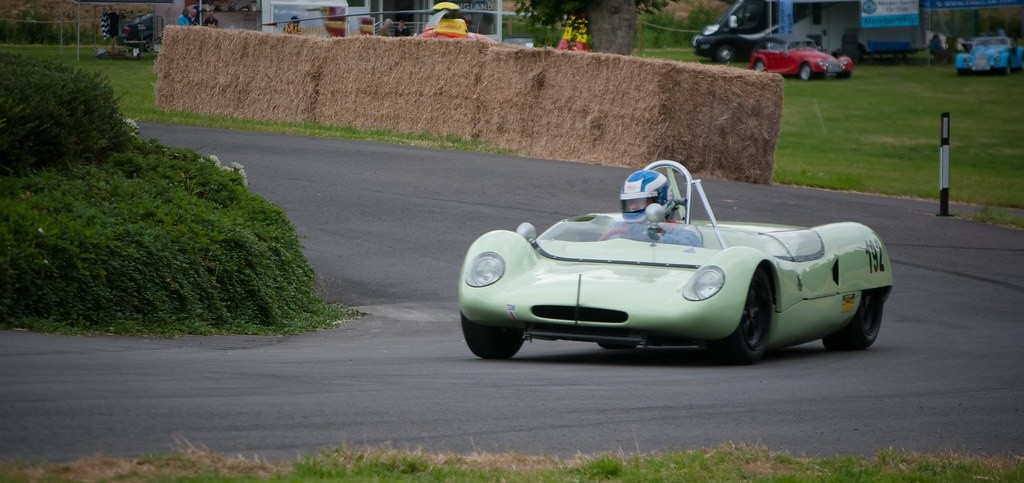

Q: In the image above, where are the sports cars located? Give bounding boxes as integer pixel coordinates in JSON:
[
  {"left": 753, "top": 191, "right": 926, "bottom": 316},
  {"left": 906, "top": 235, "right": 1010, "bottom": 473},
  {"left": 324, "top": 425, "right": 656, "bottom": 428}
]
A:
[
  {"left": 747, "top": 33, "right": 853, "bottom": 80},
  {"left": 460, "top": 159, "right": 895, "bottom": 362},
  {"left": 955, "top": 34, "right": 1024, "bottom": 76}
]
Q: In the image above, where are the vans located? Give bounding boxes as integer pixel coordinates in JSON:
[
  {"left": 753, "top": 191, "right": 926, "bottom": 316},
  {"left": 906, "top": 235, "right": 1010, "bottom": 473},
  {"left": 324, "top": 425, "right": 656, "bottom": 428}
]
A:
[{"left": 692, "top": 0, "right": 924, "bottom": 67}]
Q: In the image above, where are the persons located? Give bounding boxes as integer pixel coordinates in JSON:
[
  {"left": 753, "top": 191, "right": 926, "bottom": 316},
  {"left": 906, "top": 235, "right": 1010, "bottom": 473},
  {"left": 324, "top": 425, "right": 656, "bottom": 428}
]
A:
[
  {"left": 394, "top": 20, "right": 410, "bottom": 36},
  {"left": 283, "top": 16, "right": 301, "bottom": 35},
  {"left": 379, "top": 19, "right": 393, "bottom": 37},
  {"left": 178, "top": 8, "right": 199, "bottom": 26},
  {"left": 204, "top": 11, "right": 219, "bottom": 28},
  {"left": 127, "top": 19, "right": 139, "bottom": 57},
  {"left": 599, "top": 169, "right": 703, "bottom": 247}
]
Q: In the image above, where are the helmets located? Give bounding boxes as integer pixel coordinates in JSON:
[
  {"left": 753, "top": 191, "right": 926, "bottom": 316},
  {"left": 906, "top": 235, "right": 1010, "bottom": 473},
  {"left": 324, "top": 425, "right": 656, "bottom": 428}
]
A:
[{"left": 620, "top": 170, "right": 673, "bottom": 224}]
